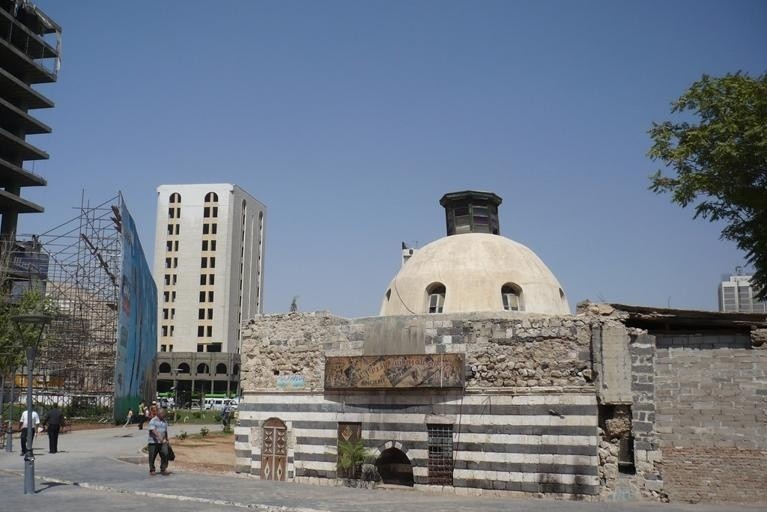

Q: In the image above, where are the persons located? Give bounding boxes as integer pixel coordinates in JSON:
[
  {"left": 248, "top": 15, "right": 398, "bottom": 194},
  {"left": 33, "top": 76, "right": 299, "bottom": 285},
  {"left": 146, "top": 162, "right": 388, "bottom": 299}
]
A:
[
  {"left": 42, "top": 402, "right": 63, "bottom": 453},
  {"left": 222, "top": 401, "right": 231, "bottom": 426},
  {"left": 124, "top": 400, "right": 167, "bottom": 430},
  {"left": 148, "top": 408, "right": 171, "bottom": 476},
  {"left": 17, "top": 404, "right": 41, "bottom": 456}
]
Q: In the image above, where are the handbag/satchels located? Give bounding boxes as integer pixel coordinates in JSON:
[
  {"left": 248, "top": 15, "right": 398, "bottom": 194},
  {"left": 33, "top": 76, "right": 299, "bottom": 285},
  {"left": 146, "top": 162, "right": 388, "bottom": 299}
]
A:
[{"left": 159, "top": 444, "right": 175, "bottom": 460}]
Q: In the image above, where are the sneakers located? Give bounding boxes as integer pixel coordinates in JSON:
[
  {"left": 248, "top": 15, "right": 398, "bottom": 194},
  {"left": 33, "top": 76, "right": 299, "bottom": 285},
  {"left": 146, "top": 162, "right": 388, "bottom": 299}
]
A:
[{"left": 150, "top": 470, "right": 156, "bottom": 475}]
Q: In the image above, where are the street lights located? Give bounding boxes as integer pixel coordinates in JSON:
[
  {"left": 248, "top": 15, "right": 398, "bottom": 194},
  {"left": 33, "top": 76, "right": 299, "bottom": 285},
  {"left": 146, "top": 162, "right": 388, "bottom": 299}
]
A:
[
  {"left": 0, "top": 344, "right": 23, "bottom": 452},
  {"left": 170, "top": 367, "right": 182, "bottom": 422},
  {"left": 8, "top": 299, "right": 54, "bottom": 495}
]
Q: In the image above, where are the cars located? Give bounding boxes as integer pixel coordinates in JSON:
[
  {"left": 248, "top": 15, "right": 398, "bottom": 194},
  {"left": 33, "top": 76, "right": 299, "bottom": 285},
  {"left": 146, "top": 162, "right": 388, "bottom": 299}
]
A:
[{"left": 158, "top": 395, "right": 239, "bottom": 412}]
